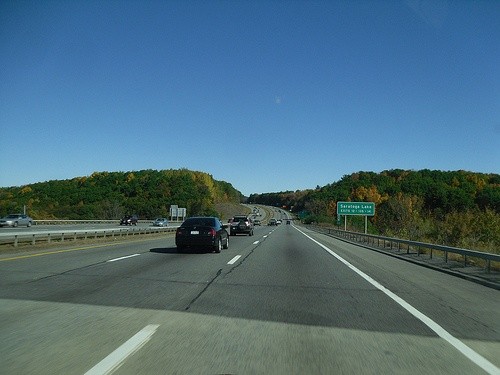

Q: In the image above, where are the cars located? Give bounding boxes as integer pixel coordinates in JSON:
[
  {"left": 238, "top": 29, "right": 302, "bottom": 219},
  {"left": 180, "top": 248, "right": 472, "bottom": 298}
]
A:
[
  {"left": 250, "top": 205, "right": 291, "bottom": 226},
  {"left": 0, "top": 213, "right": 34, "bottom": 228},
  {"left": 175, "top": 217, "right": 230, "bottom": 253},
  {"left": 153, "top": 218, "right": 168, "bottom": 228}
]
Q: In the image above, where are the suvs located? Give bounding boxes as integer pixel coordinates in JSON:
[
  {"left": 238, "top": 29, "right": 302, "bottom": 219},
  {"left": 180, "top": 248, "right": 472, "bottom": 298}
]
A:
[
  {"left": 228, "top": 215, "right": 253, "bottom": 236},
  {"left": 120, "top": 214, "right": 138, "bottom": 226}
]
